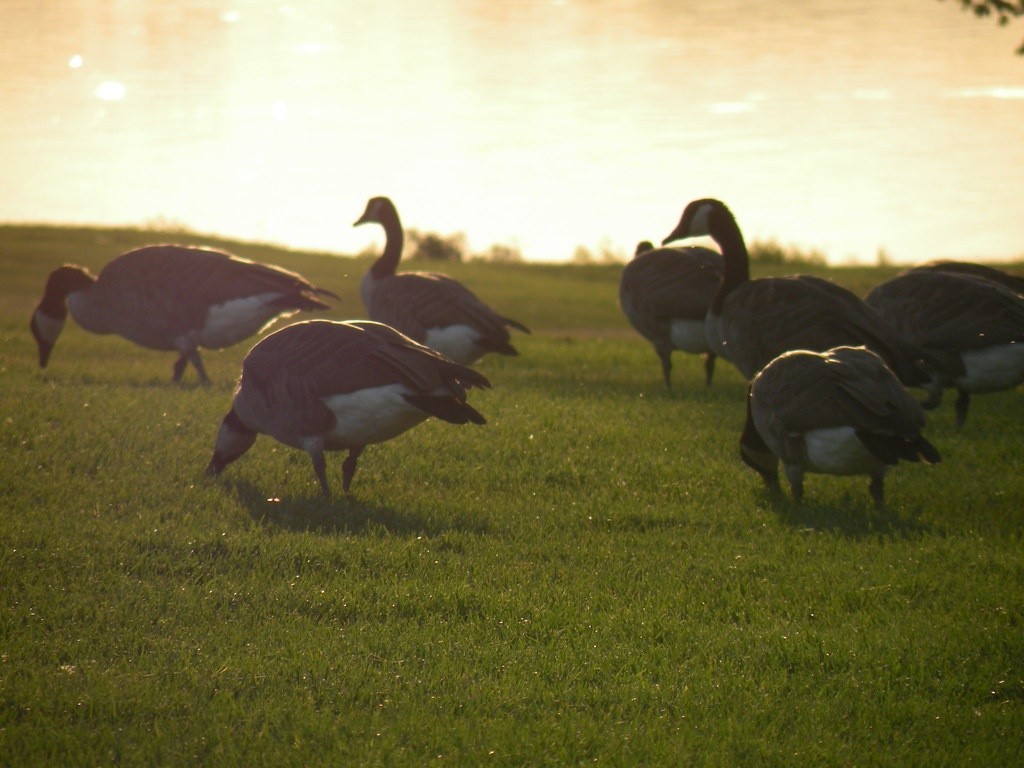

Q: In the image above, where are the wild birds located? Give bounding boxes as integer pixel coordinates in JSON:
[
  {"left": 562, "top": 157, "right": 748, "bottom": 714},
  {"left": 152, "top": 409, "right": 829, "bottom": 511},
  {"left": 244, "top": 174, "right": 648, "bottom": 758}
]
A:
[
  {"left": 614, "top": 198, "right": 1024, "bottom": 511},
  {"left": 29, "top": 242, "right": 343, "bottom": 386},
  {"left": 204, "top": 318, "right": 493, "bottom": 499},
  {"left": 354, "top": 195, "right": 534, "bottom": 370}
]
